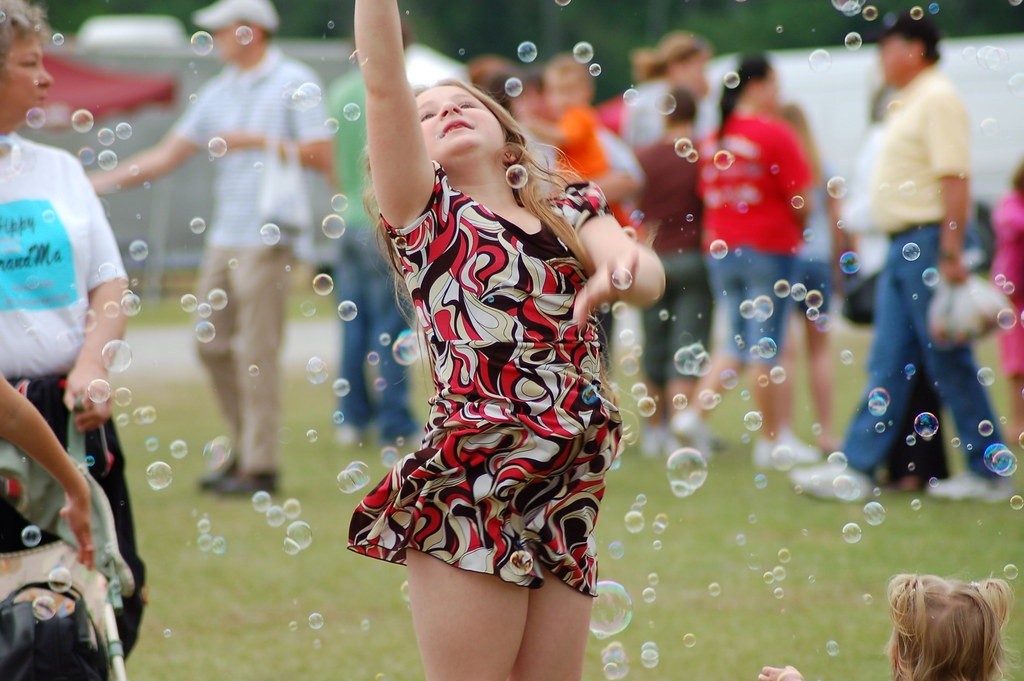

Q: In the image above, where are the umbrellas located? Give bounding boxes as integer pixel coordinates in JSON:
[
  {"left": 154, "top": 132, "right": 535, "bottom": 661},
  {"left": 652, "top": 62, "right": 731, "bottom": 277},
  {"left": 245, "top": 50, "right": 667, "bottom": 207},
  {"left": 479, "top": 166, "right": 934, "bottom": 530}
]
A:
[{"left": 40, "top": 57, "right": 173, "bottom": 126}]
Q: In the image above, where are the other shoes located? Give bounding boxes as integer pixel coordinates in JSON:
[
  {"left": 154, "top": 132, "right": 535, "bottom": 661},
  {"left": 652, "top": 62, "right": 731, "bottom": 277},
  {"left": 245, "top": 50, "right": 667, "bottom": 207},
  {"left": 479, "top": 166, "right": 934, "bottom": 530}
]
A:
[
  {"left": 671, "top": 406, "right": 711, "bottom": 458},
  {"left": 221, "top": 472, "right": 274, "bottom": 495},
  {"left": 754, "top": 428, "right": 821, "bottom": 468},
  {"left": 642, "top": 427, "right": 681, "bottom": 456},
  {"left": 707, "top": 435, "right": 728, "bottom": 453},
  {"left": 198, "top": 456, "right": 238, "bottom": 488}
]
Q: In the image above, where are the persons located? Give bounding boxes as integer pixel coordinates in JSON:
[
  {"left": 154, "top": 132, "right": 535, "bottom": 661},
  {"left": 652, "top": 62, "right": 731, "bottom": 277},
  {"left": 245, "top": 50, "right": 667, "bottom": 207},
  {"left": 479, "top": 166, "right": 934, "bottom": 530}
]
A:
[
  {"left": 467, "top": 29, "right": 841, "bottom": 465},
  {"left": 0, "top": 372, "right": 94, "bottom": 571},
  {"left": 0, "top": 1, "right": 145, "bottom": 681},
  {"left": 792, "top": 15, "right": 1024, "bottom": 503},
  {"left": 347, "top": 0, "right": 666, "bottom": 680},
  {"left": 89, "top": 1, "right": 332, "bottom": 497},
  {"left": 758, "top": 573, "right": 1011, "bottom": 681},
  {"left": 326, "top": 18, "right": 425, "bottom": 450}
]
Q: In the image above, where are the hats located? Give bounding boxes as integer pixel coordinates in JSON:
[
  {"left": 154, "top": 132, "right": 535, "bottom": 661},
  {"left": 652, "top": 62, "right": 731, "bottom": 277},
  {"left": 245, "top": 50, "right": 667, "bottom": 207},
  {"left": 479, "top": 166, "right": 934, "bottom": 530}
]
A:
[
  {"left": 860, "top": 9, "right": 942, "bottom": 44},
  {"left": 191, "top": 0, "right": 280, "bottom": 36}
]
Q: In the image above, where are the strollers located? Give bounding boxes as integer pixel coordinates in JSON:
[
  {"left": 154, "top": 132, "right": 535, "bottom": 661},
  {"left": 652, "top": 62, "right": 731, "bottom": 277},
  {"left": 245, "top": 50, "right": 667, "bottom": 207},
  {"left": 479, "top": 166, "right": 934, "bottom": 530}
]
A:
[{"left": 0, "top": 390, "right": 138, "bottom": 681}]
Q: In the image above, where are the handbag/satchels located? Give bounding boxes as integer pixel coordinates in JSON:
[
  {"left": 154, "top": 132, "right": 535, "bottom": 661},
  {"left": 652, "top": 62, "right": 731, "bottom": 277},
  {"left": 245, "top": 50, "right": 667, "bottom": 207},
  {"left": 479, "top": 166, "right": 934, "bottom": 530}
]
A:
[
  {"left": 0, "top": 395, "right": 135, "bottom": 608},
  {"left": 0, "top": 582, "right": 109, "bottom": 681},
  {"left": 928, "top": 248, "right": 1017, "bottom": 351}
]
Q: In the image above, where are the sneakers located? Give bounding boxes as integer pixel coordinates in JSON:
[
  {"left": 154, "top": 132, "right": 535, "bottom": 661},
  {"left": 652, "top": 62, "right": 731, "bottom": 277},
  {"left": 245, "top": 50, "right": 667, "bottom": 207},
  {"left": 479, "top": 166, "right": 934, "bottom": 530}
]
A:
[
  {"left": 790, "top": 462, "right": 871, "bottom": 499},
  {"left": 926, "top": 470, "right": 1013, "bottom": 503}
]
